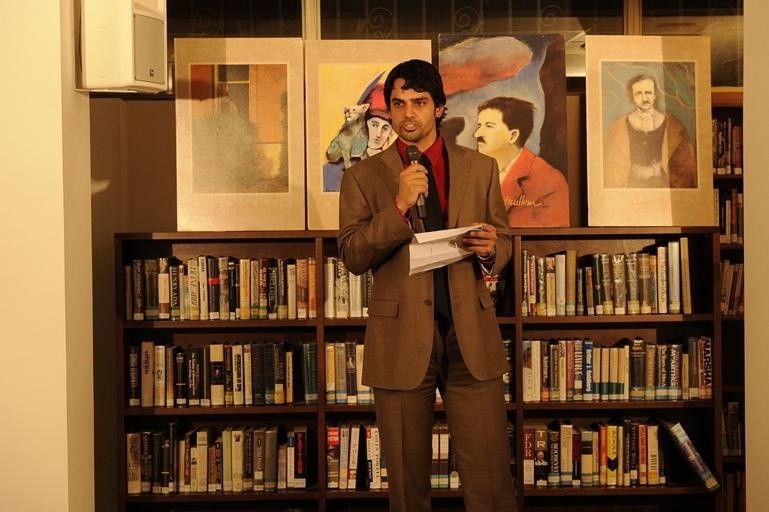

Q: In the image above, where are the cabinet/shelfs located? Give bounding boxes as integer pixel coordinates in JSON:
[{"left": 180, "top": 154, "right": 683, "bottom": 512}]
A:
[
  {"left": 711, "top": 91, "right": 744, "bottom": 511},
  {"left": 112, "top": 228, "right": 725, "bottom": 511}
]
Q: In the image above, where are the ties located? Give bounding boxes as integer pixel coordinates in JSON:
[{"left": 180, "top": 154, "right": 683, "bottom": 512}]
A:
[{"left": 413, "top": 154, "right": 451, "bottom": 319}]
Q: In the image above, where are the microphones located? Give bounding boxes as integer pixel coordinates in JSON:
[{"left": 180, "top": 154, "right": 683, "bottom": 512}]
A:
[{"left": 406, "top": 145, "right": 428, "bottom": 219}]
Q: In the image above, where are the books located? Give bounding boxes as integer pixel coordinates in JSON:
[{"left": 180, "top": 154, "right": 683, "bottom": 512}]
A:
[
  {"left": 715, "top": 188, "right": 743, "bottom": 245},
  {"left": 326, "top": 416, "right": 388, "bottom": 490},
  {"left": 124, "top": 256, "right": 316, "bottom": 320},
  {"left": 522, "top": 334, "right": 712, "bottom": 402},
  {"left": 126, "top": 339, "right": 319, "bottom": 405},
  {"left": 482, "top": 268, "right": 511, "bottom": 318},
  {"left": 507, "top": 423, "right": 520, "bottom": 489},
  {"left": 431, "top": 421, "right": 461, "bottom": 489},
  {"left": 721, "top": 403, "right": 745, "bottom": 458},
  {"left": 520, "top": 236, "right": 692, "bottom": 317},
  {"left": 523, "top": 416, "right": 719, "bottom": 496},
  {"left": 724, "top": 468, "right": 746, "bottom": 512},
  {"left": 502, "top": 339, "right": 513, "bottom": 404},
  {"left": 323, "top": 255, "right": 373, "bottom": 320},
  {"left": 326, "top": 332, "right": 375, "bottom": 405},
  {"left": 436, "top": 387, "right": 442, "bottom": 404},
  {"left": 127, "top": 418, "right": 307, "bottom": 495},
  {"left": 711, "top": 117, "right": 743, "bottom": 174},
  {"left": 719, "top": 260, "right": 744, "bottom": 317}
]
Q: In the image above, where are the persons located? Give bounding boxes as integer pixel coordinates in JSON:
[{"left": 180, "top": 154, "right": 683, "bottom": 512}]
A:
[
  {"left": 471, "top": 95, "right": 570, "bottom": 229},
  {"left": 324, "top": 84, "right": 393, "bottom": 192},
  {"left": 606, "top": 74, "right": 698, "bottom": 188},
  {"left": 337, "top": 59, "right": 518, "bottom": 512}
]
